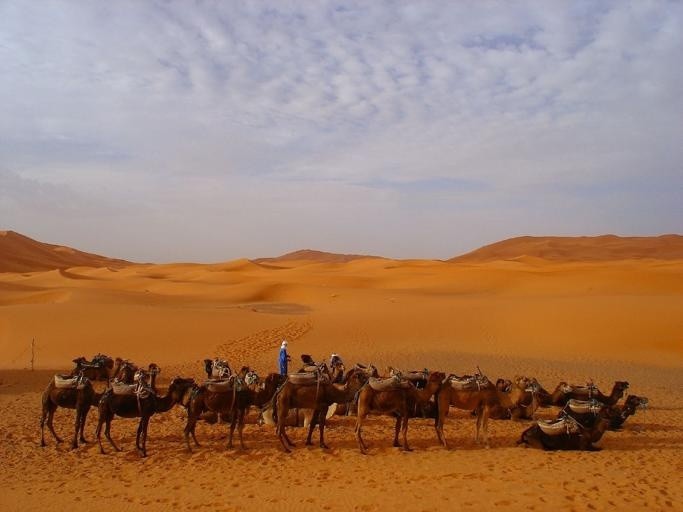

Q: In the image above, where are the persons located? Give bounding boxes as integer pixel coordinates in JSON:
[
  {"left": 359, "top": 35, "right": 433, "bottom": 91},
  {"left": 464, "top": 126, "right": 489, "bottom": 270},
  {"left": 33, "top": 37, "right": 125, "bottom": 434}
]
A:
[{"left": 279, "top": 341, "right": 291, "bottom": 376}]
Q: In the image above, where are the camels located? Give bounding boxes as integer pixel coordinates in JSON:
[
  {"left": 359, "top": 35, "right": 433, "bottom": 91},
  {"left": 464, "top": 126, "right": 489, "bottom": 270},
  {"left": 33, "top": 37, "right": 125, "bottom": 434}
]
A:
[{"left": 40, "top": 352, "right": 649, "bottom": 457}]
